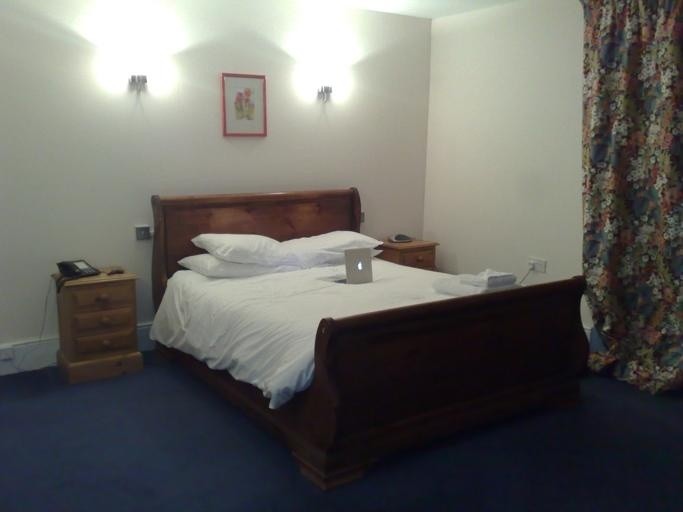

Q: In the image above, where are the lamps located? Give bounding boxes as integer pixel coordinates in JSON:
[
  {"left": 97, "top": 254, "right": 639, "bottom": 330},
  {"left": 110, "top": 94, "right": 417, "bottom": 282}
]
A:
[
  {"left": 317, "top": 85, "right": 332, "bottom": 103},
  {"left": 129, "top": 74, "right": 147, "bottom": 92}
]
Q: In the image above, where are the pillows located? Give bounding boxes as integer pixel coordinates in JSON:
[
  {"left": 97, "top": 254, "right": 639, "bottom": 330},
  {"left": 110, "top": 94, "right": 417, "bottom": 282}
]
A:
[
  {"left": 175, "top": 252, "right": 304, "bottom": 279},
  {"left": 282, "top": 230, "right": 384, "bottom": 253},
  {"left": 189, "top": 233, "right": 304, "bottom": 268},
  {"left": 294, "top": 248, "right": 384, "bottom": 267}
]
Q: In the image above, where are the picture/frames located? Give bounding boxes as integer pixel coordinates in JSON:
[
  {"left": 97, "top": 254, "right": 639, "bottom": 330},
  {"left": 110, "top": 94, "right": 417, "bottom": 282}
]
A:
[{"left": 219, "top": 72, "right": 267, "bottom": 137}]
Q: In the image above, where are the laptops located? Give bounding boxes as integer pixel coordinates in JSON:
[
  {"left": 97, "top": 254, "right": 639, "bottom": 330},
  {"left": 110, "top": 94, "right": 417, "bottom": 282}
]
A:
[{"left": 317, "top": 247, "right": 372, "bottom": 285}]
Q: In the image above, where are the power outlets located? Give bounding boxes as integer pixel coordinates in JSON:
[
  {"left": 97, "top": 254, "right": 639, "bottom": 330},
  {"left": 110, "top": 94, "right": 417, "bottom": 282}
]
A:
[
  {"left": 528, "top": 256, "right": 547, "bottom": 274},
  {"left": 0, "top": 347, "right": 15, "bottom": 360}
]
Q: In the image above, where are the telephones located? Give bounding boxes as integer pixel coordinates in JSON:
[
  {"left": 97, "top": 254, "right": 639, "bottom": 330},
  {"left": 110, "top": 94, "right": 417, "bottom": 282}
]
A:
[{"left": 57, "top": 258, "right": 102, "bottom": 279}]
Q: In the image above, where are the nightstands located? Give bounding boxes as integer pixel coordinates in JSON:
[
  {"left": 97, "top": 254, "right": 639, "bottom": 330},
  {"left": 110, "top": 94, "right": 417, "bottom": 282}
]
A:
[
  {"left": 50, "top": 265, "right": 144, "bottom": 385},
  {"left": 376, "top": 238, "right": 440, "bottom": 273}
]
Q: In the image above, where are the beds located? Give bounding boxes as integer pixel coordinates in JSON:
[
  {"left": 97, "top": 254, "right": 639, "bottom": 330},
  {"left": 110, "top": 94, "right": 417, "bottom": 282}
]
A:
[{"left": 149, "top": 186, "right": 590, "bottom": 492}]
